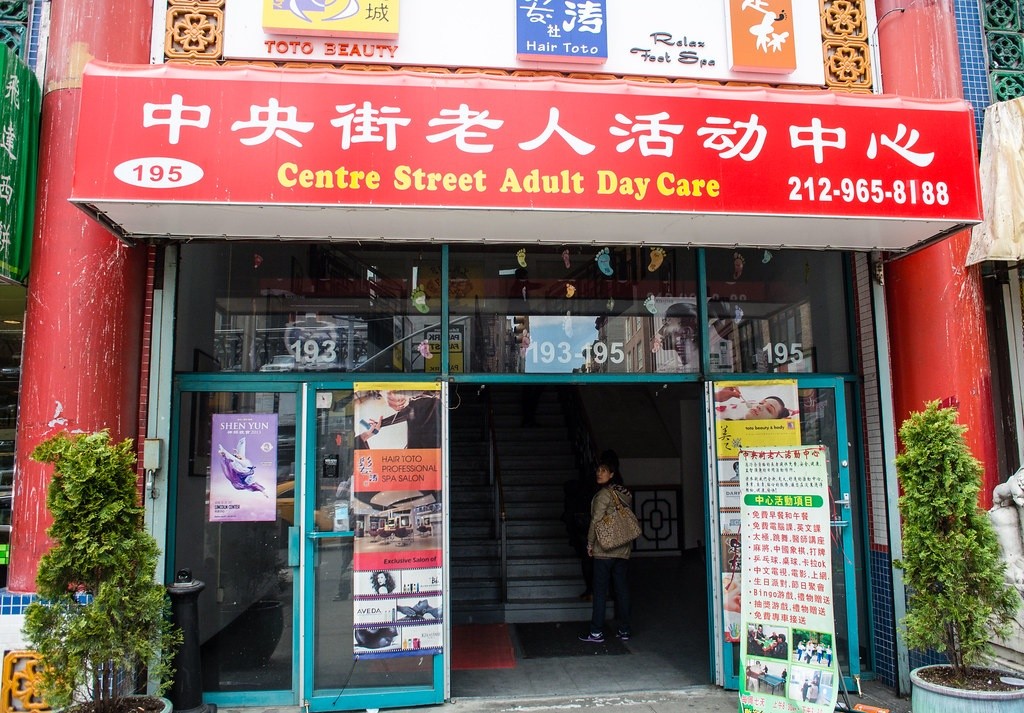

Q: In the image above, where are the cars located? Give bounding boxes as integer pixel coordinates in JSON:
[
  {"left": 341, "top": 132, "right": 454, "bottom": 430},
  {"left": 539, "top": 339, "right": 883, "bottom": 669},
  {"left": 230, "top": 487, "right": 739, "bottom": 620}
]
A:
[{"left": 256, "top": 479, "right": 341, "bottom": 549}]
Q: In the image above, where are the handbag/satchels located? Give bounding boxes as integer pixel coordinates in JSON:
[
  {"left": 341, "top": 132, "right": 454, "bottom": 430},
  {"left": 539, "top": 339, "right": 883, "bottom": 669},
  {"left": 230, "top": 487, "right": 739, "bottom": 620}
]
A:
[{"left": 594, "top": 488, "right": 642, "bottom": 552}]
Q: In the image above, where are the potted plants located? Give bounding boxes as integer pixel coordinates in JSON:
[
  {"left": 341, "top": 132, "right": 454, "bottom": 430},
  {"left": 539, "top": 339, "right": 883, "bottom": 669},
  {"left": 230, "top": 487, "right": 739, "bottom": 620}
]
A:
[
  {"left": 893, "top": 400, "right": 1024, "bottom": 713},
  {"left": 21, "top": 429, "right": 185, "bottom": 713}
]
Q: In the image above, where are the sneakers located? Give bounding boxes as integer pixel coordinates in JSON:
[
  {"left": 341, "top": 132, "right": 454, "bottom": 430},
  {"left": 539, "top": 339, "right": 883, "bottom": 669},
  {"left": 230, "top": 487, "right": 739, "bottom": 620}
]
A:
[
  {"left": 578, "top": 632, "right": 604, "bottom": 642},
  {"left": 615, "top": 630, "right": 630, "bottom": 641}
]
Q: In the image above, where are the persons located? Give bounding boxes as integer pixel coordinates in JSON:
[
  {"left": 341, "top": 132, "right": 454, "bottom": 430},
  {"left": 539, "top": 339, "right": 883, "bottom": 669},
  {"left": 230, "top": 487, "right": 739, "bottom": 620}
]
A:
[
  {"left": 797, "top": 640, "right": 832, "bottom": 668},
  {"left": 376, "top": 572, "right": 393, "bottom": 595},
  {"left": 397, "top": 599, "right": 442, "bottom": 620},
  {"left": 576, "top": 460, "right": 633, "bottom": 641},
  {"left": 800, "top": 678, "right": 820, "bottom": 703},
  {"left": 658, "top": 298, "right": 734, "bottom": 373},
  {"left": 746, "top": 395, "right": 790, "bottom": 420},
  {"left": 746, "top": 661, "right": 787, "bottom": 692},
  {"left": 354, "top": 390, "right": 442, "bottom": 450},
  {"left": 747, "top": 624, "right": 788, "bottom": 659}
]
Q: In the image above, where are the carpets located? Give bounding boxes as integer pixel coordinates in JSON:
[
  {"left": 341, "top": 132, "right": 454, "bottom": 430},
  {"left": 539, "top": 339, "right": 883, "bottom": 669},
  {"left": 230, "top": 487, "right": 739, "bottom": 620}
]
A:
[
  {"left": 512, "top": 621, "right": 633, "bottom": 659},
  {"left": 368, "top": 624, "right": 516, "bottom": 672}
]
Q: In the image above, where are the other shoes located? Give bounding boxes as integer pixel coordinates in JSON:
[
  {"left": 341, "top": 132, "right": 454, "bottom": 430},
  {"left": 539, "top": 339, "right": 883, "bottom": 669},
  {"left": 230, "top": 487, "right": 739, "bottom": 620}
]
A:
[{"left": 579, "top": 592, "right": 593, "bottom": 601}]
